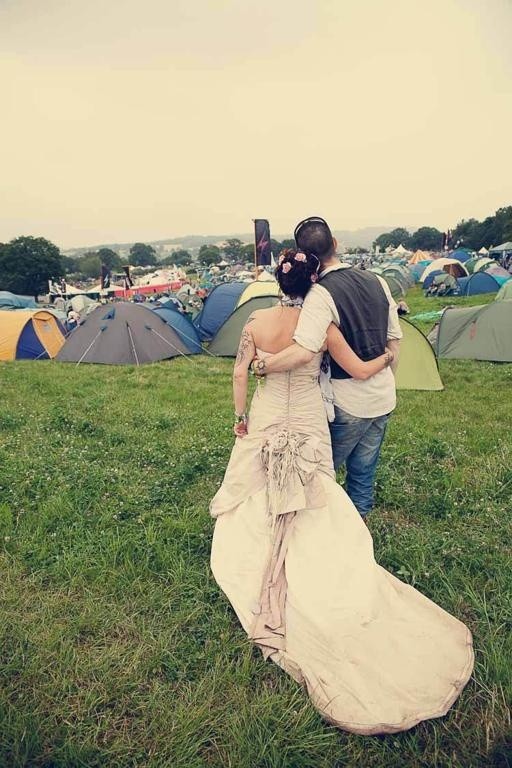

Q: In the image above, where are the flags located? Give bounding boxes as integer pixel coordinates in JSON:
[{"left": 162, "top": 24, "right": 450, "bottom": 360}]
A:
[{"left": 253, "top": 218, "right": 272, "bottom": 267}]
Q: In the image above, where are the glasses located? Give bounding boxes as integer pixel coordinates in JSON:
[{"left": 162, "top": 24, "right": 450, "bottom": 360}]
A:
[{"left": 294, "top": 217, "right": 327, "bottom": 245}]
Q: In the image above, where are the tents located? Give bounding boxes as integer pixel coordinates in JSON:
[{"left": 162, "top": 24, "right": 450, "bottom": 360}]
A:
[{"left": 1, "top": 242, "right": 511, "bottom": 393}]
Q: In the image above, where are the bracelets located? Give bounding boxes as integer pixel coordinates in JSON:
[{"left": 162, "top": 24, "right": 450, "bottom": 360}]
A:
[
  {"left": 248, "top": 358, "right": 264, "bottom": 379},
  {"left": 233, "top": 410, "right": 249, "bottom": 423}
]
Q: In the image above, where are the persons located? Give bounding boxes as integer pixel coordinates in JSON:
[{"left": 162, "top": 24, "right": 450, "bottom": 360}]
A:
[
  {"left": 247, "top": 215, "right": 408, "bottom": 521},
  {"left": 232, "top": 248, "right": 395, "bottom": 558}
]
